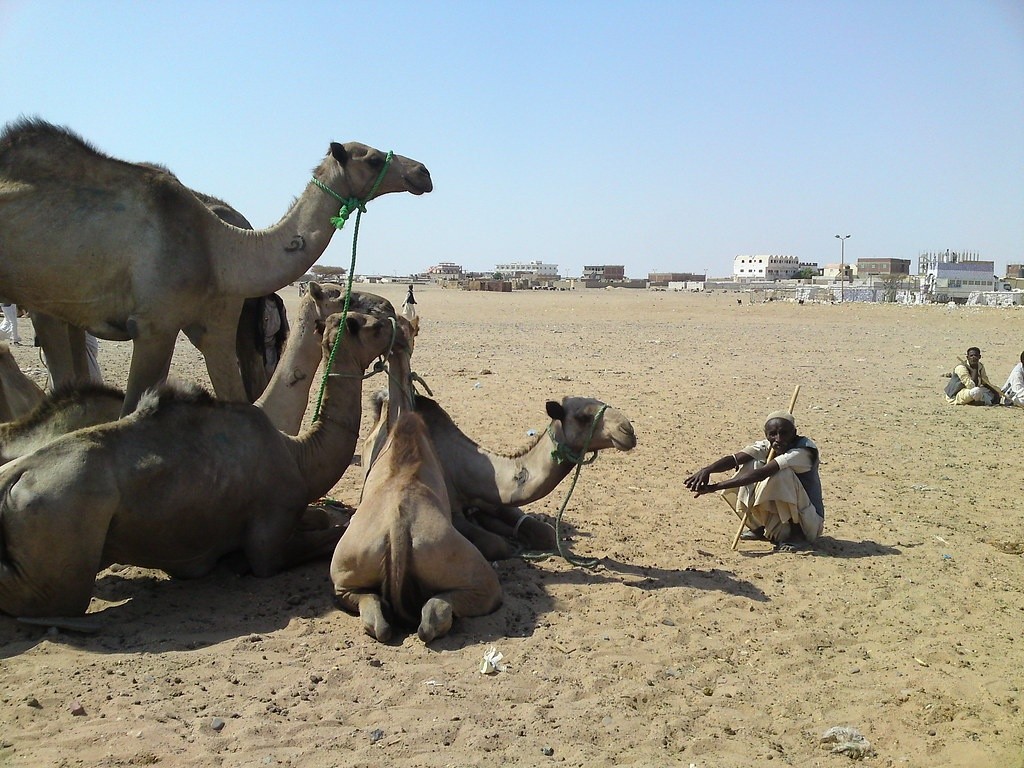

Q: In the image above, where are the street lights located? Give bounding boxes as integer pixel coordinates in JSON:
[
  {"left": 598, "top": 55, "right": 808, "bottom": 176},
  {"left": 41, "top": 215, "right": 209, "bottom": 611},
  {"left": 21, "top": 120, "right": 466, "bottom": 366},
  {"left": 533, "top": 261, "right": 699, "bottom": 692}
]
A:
[{"left": 835, "top": 235, "right": 851, "bottom": 301}]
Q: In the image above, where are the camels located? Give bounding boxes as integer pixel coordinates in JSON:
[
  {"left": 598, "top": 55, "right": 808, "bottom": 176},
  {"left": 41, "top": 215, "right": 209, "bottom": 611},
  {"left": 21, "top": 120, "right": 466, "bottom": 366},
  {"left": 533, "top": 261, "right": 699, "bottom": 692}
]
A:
[{"left": 0, "top": 117, "right": 637, "bottom": 640}]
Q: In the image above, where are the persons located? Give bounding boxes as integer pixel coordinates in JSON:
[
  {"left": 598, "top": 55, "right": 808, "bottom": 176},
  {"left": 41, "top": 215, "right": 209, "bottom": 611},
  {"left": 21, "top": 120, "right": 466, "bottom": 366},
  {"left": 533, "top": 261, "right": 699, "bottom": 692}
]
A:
[
  {"left": 1002, "top": 351, "right": 1024, "bottom": 405},
  {"left": 235, "top": 293, "right": 290, "bottom": 404},
  {"left": 944, "top": 347, "right": 1001, "bottom": 405},
  {"left": 684, "top": 411, "right": 825, "bottom": 551},
  {"left": 401, "top": 284, "right": 417, "bottom": 317},
  {"left": 0, "top": 303, "right": 23, "bottom": 346}
]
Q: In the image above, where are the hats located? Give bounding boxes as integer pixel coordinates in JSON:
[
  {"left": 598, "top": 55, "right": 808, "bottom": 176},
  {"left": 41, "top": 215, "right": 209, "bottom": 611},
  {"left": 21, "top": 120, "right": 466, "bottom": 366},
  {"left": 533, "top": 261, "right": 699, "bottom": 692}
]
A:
[{"left": 764, "top": 411, "right": 794, "bottom": 426}]
